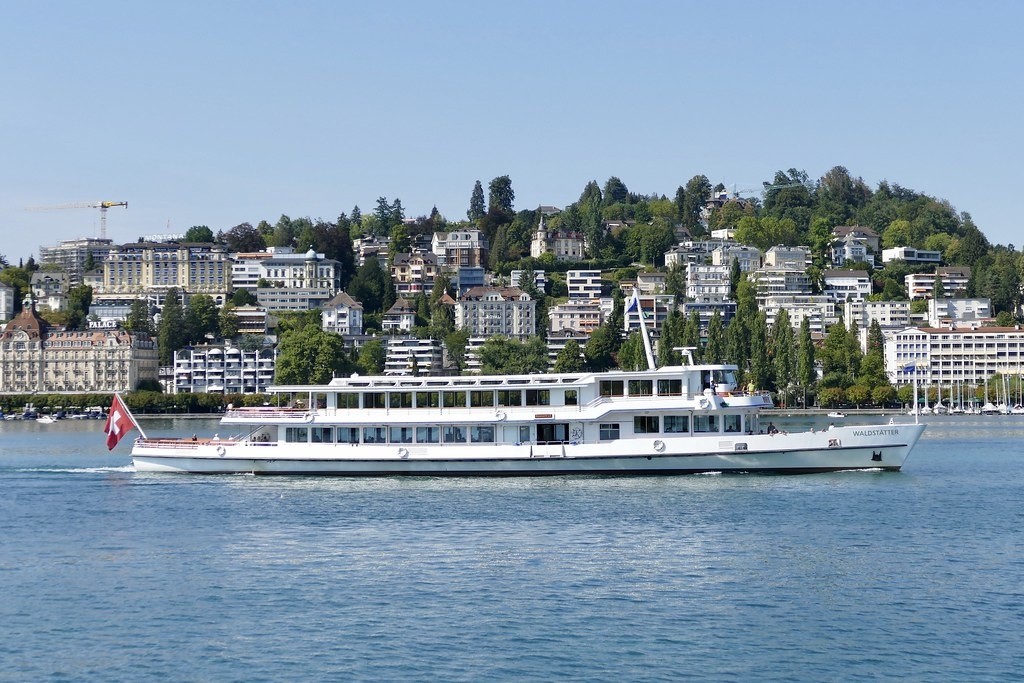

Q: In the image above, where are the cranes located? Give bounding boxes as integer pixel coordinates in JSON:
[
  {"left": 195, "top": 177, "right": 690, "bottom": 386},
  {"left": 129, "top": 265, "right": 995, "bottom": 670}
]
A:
[{"left": 22, "top": 199, "right": 128, "bottom": 259}]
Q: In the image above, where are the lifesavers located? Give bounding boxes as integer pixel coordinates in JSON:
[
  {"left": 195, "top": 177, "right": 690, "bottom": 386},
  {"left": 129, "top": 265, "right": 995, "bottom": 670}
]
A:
[
  {"left": 653, "top": 439, "right": 663, "bottom": 450},
  {"left": 304, "top": 412, "right": 314, "bottom": 423},
  {"left": 495, "top": 411, "right": 506, "bottom": 421},
  {"left": 571, "top": 428, "right": 582, "bottom": 439},
  {"left": 397, "top": 447, "right": 407, "bottom": 457},
  {"left": 216, "top": 445, "right": 225, "bottom": 455},
  {"left": 697, "top": 397, "right": 709, "bottom": 409}
]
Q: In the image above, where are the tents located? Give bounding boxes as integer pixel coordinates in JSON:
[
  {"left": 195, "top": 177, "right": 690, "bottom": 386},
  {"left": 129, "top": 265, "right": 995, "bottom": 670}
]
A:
[{"left": 917, "top": 397, "right": 1003, "bottom": 408}]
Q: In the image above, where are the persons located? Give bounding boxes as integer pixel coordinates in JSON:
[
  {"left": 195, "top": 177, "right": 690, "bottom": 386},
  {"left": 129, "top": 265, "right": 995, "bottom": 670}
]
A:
[
  {"left": 192, "top": 421, "right": 836, "bottom": 448},
  {"left": 295, "top": 379, "right": 755, "bottom": 407}
]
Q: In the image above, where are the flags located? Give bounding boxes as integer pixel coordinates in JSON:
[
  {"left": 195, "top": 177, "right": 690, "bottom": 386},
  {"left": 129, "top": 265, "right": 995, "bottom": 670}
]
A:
[
  {"left": 905, "top": 361, "right": 915, "bottom": 372},
  {"left": 104, "top": 395, "right": 136, "bottom": 451}
]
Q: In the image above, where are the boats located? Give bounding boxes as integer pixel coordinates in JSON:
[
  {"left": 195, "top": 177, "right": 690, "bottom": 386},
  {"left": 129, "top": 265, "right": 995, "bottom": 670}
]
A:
[
  {"left": 129, "top": 285, "right": 928, "bottom": 472},
  {"left": 827, "top": 410, "right": 846, "bottom": 418},
  {"left": 36, "top": 416, "right": 58, "bottom": 424}
]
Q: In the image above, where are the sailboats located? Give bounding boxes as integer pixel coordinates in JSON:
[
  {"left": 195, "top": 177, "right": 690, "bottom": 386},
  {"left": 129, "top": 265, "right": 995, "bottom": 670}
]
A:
[{"left": 906, "top": 366, "right": 1024, "bottom": 415}]
